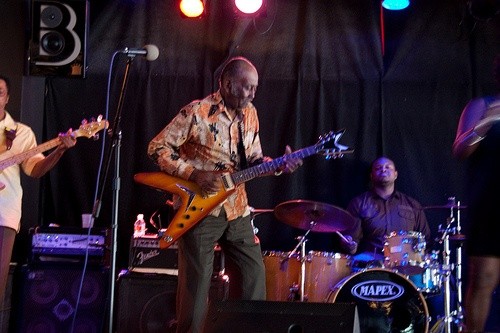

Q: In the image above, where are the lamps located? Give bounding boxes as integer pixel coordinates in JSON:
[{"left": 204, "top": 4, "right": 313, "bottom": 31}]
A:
[{"left": 180, "top": 0, "right": 206, "bottom": 19}]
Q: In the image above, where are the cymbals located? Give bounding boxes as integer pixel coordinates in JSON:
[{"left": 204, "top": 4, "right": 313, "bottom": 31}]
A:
[{"left": 274, "top": 200, "right": 356, "bottom": 232}]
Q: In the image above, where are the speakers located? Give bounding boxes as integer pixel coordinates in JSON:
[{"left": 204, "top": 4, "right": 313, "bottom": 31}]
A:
[
  {"left": 10, "top": 260, "right": 106, "bottom": 333},
  {"left": 115, "top": 274, "right": 178, "bottom": 333},
  {"left": 25, "top": 0, "right": 89, "bottom": 78},
  {"left": 213, "top": 298, "right": 361, "bottom": 333}
]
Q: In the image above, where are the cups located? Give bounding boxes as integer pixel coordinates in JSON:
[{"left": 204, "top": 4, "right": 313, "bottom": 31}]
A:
[{"left": 81, "top": 214, "right": 92, "bottom": 229}]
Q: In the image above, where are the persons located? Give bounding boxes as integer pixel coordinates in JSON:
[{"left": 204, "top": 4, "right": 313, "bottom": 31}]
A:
[
  {"left": 0, "top": 78, "right": 76, "bottom": 306},
  {"left": 453, "top": 98, "right": 500, "bottom": 333},
  {"left": 148, "top": 57, "right": 303, "bottom": 333},
  {"left": 340, "top": 158, "right": 430, "bottom": 253}
]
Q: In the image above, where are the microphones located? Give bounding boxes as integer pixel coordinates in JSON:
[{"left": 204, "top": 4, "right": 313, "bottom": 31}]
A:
[{"left": 122, "top": 44, "right": 160, "bottom": 61}]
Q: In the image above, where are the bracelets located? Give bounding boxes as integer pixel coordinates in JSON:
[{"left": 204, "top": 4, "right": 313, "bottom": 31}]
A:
[{"left": 461, "top": 129, "right": 485, "bottom": 146}]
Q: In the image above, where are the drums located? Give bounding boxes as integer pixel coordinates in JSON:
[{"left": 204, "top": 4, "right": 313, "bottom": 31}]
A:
[
  {"left": 406, "top": 254, "right": 442, "bottom": 294},
  {"left": 326, "top": 269, "right": 429, "bottom": 333},
  {"left": 301, "top": 251, "right": 352, "bottom": 302},
  {"left": 382, "top": 230, "right": 426, "bottom": 274},
  {"left": 261, "top": 250, "right": 301, "bottom": 302}
]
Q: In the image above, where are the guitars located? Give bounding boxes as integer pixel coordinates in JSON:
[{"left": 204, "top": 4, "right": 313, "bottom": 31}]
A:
[
  {"left": 133, "top": 128, "right": 355, "bottom": 250},
  {"left": 0, "top": 114, "right": 109, "bottom": 190}
]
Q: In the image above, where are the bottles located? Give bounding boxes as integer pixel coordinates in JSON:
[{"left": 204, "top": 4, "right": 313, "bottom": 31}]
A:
[{"left": 133, "top": 214, "right": 146, "bottom": 239}]
